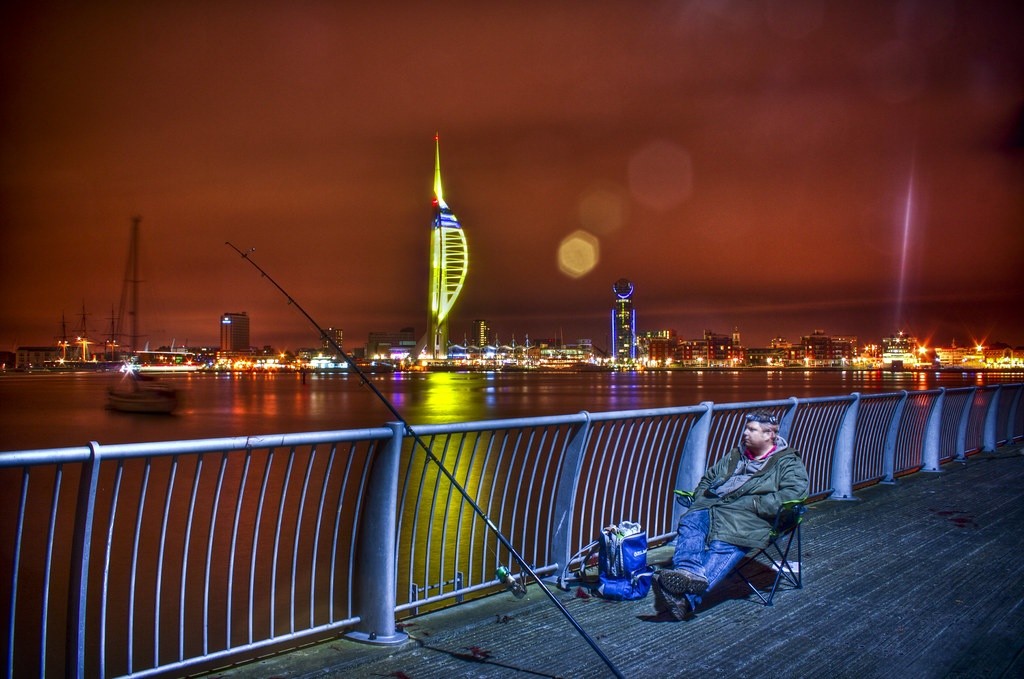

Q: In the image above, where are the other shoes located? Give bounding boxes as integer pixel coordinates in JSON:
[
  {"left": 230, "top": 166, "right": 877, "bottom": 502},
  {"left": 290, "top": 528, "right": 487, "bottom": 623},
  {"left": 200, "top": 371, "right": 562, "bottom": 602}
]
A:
[
  {"left": 653, "top": 574, "right": 691, "bottom": 620},
  {"left": 661, "top": 570, "right": 710, "bottom": 593}
]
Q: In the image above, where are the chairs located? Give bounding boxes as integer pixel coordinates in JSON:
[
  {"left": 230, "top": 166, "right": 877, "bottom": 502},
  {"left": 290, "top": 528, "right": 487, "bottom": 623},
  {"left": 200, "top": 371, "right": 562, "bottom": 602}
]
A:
[{"left": 673, "top": 480, "right": 808, "bottom": 605}]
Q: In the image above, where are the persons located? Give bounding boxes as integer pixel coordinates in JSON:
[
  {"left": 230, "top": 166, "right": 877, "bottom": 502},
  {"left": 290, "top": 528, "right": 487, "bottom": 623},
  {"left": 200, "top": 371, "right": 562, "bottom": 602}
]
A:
[{"left": 651, "top": 410, "right": 810, "bottom": 621}]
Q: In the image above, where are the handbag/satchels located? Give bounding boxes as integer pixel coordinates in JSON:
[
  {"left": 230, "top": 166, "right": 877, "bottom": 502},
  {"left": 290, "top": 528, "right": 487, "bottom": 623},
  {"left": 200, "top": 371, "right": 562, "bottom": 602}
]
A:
[{"left": 595, "top": 526, "right": 653, "bottom": 599}]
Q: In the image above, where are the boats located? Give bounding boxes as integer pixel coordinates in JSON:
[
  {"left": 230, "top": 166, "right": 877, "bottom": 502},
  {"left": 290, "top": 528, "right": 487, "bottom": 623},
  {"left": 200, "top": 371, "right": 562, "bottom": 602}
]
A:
[
  {"left": 106, "top": 365, "right": 184, "bottom": 413},
  {"left": 41, "top": 350, "right": 660, "bottom": 372}
]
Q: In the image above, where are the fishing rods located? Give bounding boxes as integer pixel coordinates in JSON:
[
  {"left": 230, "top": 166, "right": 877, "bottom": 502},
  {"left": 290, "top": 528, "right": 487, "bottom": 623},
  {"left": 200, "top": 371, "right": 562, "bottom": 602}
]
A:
[{"left": 223, "top": 240, "right": 626, "bottom": 678}]
